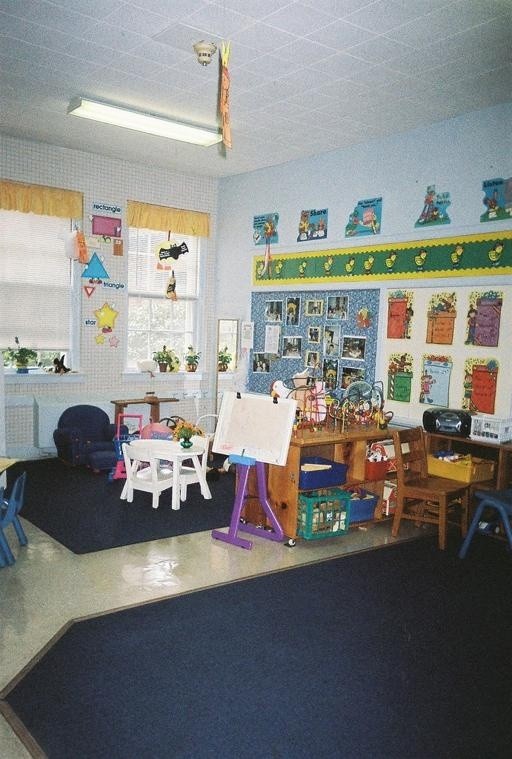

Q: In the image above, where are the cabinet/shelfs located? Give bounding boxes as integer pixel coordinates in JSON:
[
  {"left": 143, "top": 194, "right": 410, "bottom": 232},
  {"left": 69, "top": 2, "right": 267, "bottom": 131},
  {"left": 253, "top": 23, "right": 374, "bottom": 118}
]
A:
[
  {"left": 420, "top": 427, "right": 512, "bottom": 543},
  {"left": 234, "top": 420, "right": 421, "bottom": 547}
]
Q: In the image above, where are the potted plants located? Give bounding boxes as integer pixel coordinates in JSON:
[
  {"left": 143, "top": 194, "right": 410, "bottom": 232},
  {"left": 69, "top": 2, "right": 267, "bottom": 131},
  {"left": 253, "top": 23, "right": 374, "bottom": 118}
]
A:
[{"left": 4, "top": 336, "right": 38, "bottom": 374}]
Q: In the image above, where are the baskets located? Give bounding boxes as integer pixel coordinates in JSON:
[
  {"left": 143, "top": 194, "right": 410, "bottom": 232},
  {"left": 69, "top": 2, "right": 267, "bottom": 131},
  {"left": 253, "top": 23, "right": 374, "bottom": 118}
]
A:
[{"left": 296, "top": 486, "right": 352, "bottom": 541}]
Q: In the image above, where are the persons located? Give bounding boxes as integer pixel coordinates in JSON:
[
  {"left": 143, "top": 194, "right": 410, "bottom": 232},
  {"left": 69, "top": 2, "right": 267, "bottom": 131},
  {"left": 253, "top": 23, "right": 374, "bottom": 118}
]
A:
[
  {"left": 256, "top": 361, "right": 267, "bottom": 371},
  {"left": 394, "top": 356, "right": 411, "bottom": 372},
  {"left": 312, "top": 328, "right": 318, "bottom": 341},
  {"left": 435, "top": 293, "right": 454, "bottom": 315},
  {"left": 463, "top": 370, "right": 473, "bottom": 408},
  {"left": 329, "top": 304, "right": 346, "bottom": 319},
  {"left": 464, "top": 304, "right": 478, "bottom": 345},
  {"left": 402, "top": 303, "right": 415, "bottom": 338},
  {"left": 422, "top": 369, "right": 434, "bottom": 403}
]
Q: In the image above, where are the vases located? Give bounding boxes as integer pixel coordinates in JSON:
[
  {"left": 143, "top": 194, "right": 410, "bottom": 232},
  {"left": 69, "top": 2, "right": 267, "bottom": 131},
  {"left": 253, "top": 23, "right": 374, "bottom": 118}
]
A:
[
  {"left": 159, "top": 362, "right": 167, "bottom": 372},
  {"left": 218, "top": 363, "right": 228, "bottom": 372},
  {"left": 180, "top": 437, "right": 193, "bottom": 449},
  {"left": 187, "top": 364, "right": 197, "bottom": 372}
]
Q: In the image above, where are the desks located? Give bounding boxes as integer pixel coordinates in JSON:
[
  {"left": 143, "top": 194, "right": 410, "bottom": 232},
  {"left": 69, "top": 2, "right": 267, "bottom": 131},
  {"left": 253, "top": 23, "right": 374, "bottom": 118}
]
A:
[
  {"left": 0, "top": 457, "right": 20, "bottom": 477},
  {"left": 112, "top": 397, "right": 181, "bottom": 425},
  {"left": 129, "top": 438, "right": 213, "bottom": 511}
]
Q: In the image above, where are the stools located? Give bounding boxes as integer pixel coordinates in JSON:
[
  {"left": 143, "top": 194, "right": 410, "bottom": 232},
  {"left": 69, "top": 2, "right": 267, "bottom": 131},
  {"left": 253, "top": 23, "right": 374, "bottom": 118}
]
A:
[
  {"left": 87, "top": 450, "right": 117, "bottom": 472},
  {"left": 458, "top": 488, "right": 511, "bottom": 561}
]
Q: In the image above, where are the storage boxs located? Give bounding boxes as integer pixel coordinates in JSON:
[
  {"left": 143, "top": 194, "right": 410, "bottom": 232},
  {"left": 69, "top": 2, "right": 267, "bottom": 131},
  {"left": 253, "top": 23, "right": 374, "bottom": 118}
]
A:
[
  {"left": 383, "top": 480, "right": 397, "bottom": 513},
  {"left": 367, "top": 457, "right": 387, "bottom": 482},
  {"left": 374, "top": 440, "right": 409, "bottom": 473},
  {"left": 298, "top": 456, "right": 349, "bottom": 490},
  {"left": 338, "top": 488, "right": 378, "bottom": 522},
  {"left": 427, "top": 451, "right": 496, "bottom": 484}
]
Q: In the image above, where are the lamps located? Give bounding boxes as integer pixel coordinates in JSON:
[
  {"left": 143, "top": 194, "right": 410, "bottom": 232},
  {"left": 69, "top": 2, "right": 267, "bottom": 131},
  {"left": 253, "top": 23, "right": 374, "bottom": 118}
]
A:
[{"left": 66, "top": 94, "right": 223, "bottom": 148}]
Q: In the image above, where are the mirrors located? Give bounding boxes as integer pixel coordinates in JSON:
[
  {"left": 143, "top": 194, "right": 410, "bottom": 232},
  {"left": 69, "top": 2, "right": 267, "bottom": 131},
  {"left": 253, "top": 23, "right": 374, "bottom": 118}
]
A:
[{"left": 215, "top": 318, "right": 239, "bottom": 432}]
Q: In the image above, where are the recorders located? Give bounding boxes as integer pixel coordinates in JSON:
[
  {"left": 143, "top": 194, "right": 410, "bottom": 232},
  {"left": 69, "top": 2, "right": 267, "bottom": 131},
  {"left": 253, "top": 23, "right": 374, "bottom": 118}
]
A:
[{"left": 422, "top": 408, "right": 471, "bottom": 436}]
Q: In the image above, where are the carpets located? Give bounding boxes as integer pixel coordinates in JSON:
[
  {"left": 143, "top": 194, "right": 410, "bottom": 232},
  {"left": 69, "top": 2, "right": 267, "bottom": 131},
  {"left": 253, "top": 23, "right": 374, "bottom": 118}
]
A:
[
  {"left": 0, "top": 456, "right": 236, "bottom": 556},
  {"left": 1, "top": 531, "right": 510, "bottom": 759}
]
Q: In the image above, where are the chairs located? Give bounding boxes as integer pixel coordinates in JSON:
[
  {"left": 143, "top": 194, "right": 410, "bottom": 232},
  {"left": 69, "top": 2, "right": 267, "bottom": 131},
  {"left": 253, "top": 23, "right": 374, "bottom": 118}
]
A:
[
  {"left": 171, "top": 435, "right": 211, "bottom": 501},
  {"left": 52, "top": 404, "right": 130, "bottom": 467},
  {"left": 391, "top": 425, "right": 470, "bottom": 551},
  {"left": 0, "top": 471, "right": 28, "bottom": 566},
  {"left": 121, "top": 443, "right": 174, "bottom": 509}
]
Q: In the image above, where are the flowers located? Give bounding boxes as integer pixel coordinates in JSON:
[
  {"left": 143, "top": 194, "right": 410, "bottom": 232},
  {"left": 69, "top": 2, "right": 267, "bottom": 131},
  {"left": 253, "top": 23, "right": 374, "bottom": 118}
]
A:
[
  {"left": 152, "top": 344, "right": 181, "bottom": 373},
  {"left": 218, "top": 346, "right": 232, "bottom": 364},
  {"left": 172, "top": 420, "right": 205, "bottom": 442},
  {"left": 184, "top": 345, "right": 201, "bottom": 364}
]
